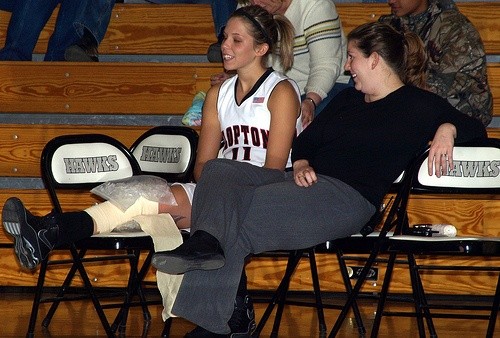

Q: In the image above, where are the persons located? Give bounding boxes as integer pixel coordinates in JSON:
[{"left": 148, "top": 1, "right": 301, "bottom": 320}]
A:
[
  {"left": 149, "top": 22, "right": 490, "bottom": 338},
  {"left": 343, "top": 0, "right": 493, "bottom": 127},
  {"left": 0, "top": 4, "right": 302, "bottom": 338},
  {"left": 247, "top": 0, "right": 348, "bottom": 129},
  {"left": 64, "top": 0, "right": 239, "bottom": 63},
  {"left": 0, "top": 0, "right": 88, "bottom": 60}
]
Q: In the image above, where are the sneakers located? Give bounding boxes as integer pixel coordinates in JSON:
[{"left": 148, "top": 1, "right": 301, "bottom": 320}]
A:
[
  {"left": 1, "top": 197, "right": 62, "bottom": 272},
  {"left": 227, "top": 294, "right": 257, "bottom": 338}
]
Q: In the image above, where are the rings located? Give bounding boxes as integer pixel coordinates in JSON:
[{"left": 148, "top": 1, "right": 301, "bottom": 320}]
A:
[
  {"left": 441, "top": 154, "right": 446, "bottom": 156},
  {"left": 298, "top": 174, "right": 306, "bottom": 180}
]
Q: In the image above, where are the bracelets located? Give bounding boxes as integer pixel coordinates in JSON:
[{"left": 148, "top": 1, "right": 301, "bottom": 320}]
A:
[{"left": 303, "top": 96, "right": 317, "bottom": 111}]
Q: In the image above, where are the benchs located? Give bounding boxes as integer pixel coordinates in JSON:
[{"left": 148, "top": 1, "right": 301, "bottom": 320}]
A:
[{"left": 0, "top": 4, "right": 500, "bottom": 299}]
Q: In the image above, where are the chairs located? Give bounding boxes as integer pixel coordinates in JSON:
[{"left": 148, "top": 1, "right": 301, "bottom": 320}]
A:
[{"left": 26, "top": 125, "right": 500, "bottom": 338}]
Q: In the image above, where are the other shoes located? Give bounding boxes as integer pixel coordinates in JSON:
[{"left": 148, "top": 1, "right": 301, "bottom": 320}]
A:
[
  {"left": 62, "top": 39, "right": 99, "bottom": 63},
  {"left": 206, "top": 41, "right": 223, "bottom": 63},
  {"left": 151, "top": 230, "right": 225, "bottom": 276},
  {"left": 183, "top": 325, "right": 231, "bottom": 338}
]
